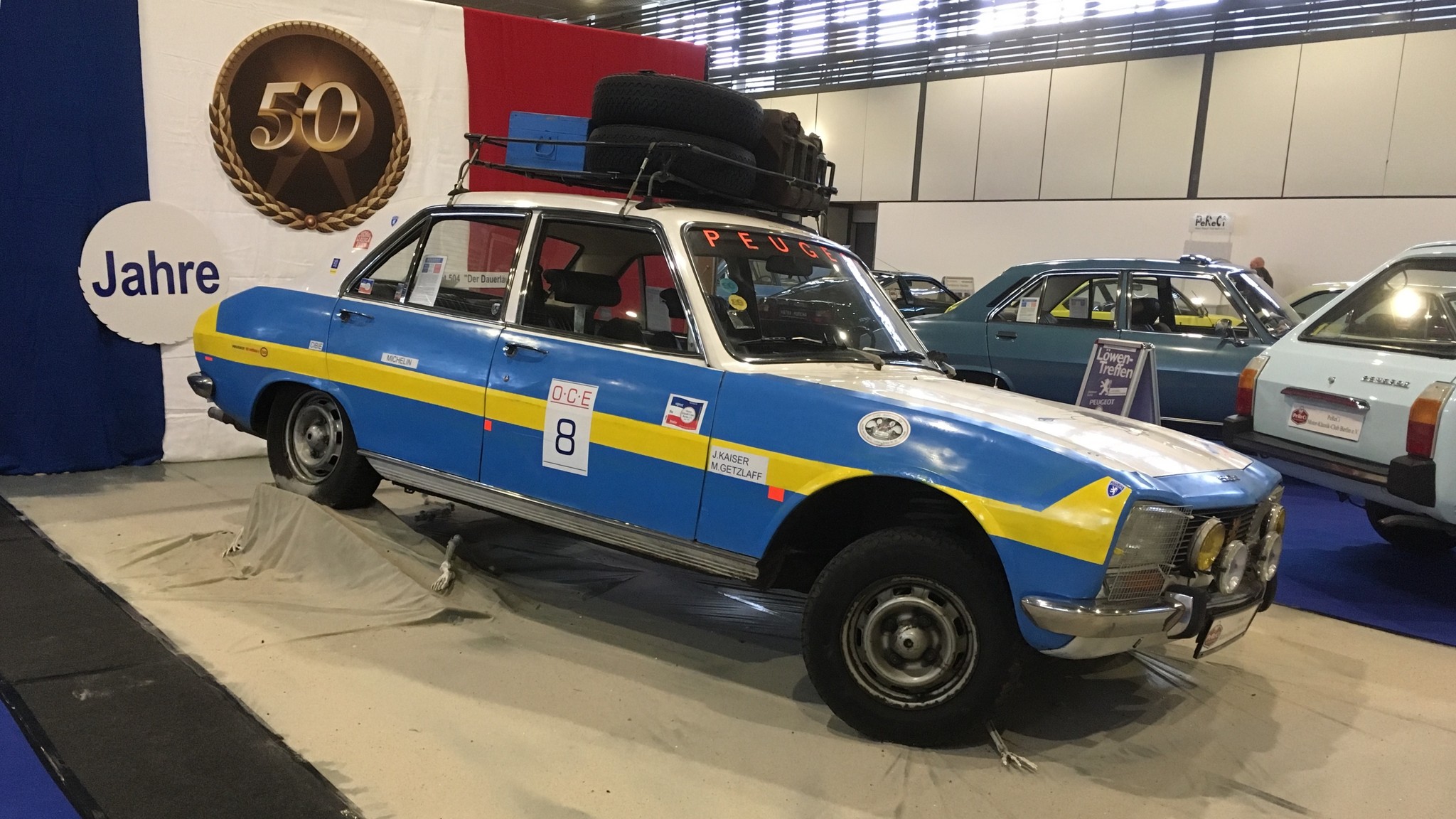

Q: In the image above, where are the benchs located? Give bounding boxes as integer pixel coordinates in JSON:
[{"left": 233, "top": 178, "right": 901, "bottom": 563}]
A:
[{"left": 435, "top": 294, "right": 598, "bottom": 338}]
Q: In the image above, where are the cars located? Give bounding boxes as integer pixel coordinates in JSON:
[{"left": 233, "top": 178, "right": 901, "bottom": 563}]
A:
[
  {"left": 1221, "top": 242, "right": 1456, "bottom": 547},
  {"left": 947, "top": 276, "right": 1244, "bottom": 330},
  {"left": 757, "top": 271, "right": 965, "bottom": 339},
  {"left": 861, "top": 257, "right": 1304, "bottom": 439},
  {"left": 1288, "top": 281, "right": 1455, "bottom": 340},
  {"left": 186, "top": 133, "right": 1271, "bottom": 751}
]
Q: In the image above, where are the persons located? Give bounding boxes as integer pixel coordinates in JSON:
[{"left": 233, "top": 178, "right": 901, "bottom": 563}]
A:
[{"left": 1249, "top": 257, "right": 1274, "bottom": 289}]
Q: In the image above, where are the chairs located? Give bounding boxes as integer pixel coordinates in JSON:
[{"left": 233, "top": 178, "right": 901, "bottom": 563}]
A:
[
  {"left": 660, "top": 288, "right": 731, "bottom": 360},
  {"left": 1129, "top": 297, "right": 1173, "bottom": 333},
  {"left": 543, "top": 269, "right": 622, "bottom": 336}
]
